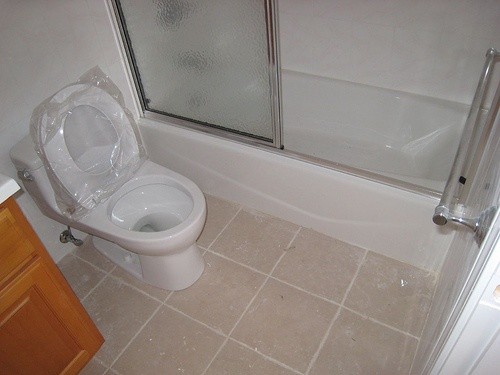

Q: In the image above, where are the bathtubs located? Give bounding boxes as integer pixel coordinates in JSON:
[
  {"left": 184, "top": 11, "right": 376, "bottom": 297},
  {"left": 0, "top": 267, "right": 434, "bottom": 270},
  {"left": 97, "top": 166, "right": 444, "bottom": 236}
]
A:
[{"left": 141, "top": 63, "right": 481, "bottom": 274}]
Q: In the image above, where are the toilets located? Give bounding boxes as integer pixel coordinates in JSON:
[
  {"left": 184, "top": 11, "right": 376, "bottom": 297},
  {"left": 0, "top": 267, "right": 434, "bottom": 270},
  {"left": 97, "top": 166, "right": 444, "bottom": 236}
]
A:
[{"left": 9, "top": 65, "right": 207, "bottom": 291}]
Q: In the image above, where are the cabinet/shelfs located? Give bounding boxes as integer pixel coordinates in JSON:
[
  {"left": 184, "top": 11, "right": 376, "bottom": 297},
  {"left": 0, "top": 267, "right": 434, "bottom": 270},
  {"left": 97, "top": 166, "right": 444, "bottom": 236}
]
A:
[{"left": 1, "top": 197, "right": 105, "bottom": 375}]
